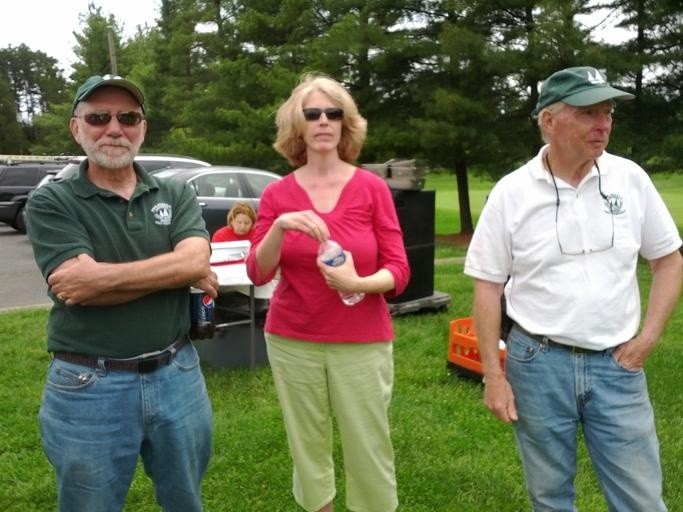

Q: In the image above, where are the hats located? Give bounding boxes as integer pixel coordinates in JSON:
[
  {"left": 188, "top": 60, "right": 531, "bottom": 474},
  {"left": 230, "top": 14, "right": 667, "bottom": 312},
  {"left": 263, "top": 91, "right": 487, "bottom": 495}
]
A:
[
  {"left": 72, "top": 73, "right": 145, "bottom": 113},
  {"left": 536, "top": 66, "right": 636, "bottom": 114}
]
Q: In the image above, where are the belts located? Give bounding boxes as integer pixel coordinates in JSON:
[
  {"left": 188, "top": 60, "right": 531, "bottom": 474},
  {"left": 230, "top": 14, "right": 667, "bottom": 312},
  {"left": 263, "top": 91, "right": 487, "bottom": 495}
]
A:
[
  {"left": 53, "top": 334, "right": 189, "bottom": 375},
  {"left": 512, "top": 320, "right": 607, "bottom": 355}
]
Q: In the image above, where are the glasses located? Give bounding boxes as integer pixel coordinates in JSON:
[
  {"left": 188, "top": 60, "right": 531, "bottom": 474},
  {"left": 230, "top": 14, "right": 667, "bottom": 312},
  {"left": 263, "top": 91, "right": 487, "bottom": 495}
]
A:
[
  {"left": 555, "top": 198, "right": 615, "bottom": 256},
  {"left": 303, "top": 107, "right": 344, "bottom": 121},
  {"left": 72, "top": 110, "right": 145, "bottom": 127}
]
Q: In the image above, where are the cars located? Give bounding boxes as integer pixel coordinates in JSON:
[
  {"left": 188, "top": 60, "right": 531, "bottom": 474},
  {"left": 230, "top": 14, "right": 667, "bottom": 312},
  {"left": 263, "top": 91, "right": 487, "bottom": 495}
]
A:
[{"left": 0, "top": 155, "right": 284, "bottom": 242}]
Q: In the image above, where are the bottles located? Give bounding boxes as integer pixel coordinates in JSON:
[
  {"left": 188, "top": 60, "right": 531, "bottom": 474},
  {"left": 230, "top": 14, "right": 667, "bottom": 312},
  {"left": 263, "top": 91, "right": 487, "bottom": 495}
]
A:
[
  {"left": 188, "top": 286, "right": 217, "bottom": 342},
  {"left": 317, "top": 238, "right": 366, "bottom": 307}
]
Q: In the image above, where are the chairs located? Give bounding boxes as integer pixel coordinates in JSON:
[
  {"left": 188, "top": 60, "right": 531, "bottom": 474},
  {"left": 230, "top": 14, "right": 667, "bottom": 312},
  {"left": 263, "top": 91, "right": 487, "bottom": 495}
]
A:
[{"left": 196, "top": 182, "right": 239, "bottom": 197}]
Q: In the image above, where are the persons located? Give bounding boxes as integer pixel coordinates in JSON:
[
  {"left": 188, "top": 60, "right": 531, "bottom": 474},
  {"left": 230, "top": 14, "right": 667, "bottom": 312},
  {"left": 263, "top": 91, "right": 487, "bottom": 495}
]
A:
[
  {"left": 212, "top": 201, "right": 257, "bottom": 242},
  {"left": 25, "top": 74, "right": 220, "bottom": 512},
  {"left": 462, "top": 65, "right": 683, "bottom": 512},
  {"left": 245, "top": 69, "right": 412, "bottom": 512}
]
